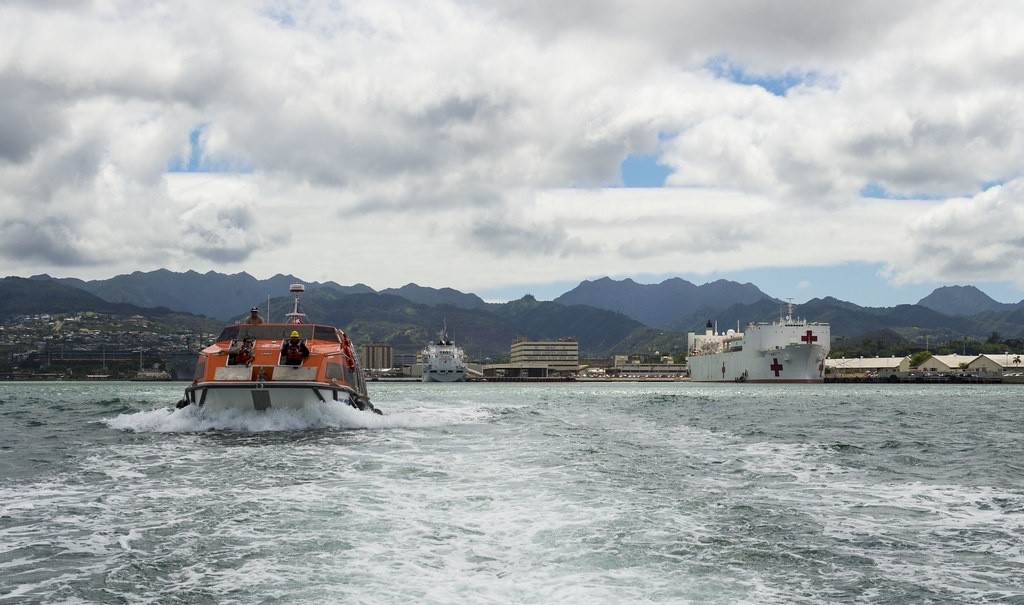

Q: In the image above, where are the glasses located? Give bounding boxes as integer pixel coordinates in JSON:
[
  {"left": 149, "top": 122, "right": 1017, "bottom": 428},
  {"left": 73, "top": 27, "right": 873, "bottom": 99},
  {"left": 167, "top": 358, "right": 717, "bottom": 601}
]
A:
[{"left": 246, "top": 341, "right": 252, "bottom": 343}]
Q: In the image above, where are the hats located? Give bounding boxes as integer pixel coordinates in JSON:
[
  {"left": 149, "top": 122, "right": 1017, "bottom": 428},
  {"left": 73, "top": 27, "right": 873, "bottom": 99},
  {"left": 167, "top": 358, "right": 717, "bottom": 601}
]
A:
[{"left": 251, "top": 307, "right": 258, "bottom": 311}]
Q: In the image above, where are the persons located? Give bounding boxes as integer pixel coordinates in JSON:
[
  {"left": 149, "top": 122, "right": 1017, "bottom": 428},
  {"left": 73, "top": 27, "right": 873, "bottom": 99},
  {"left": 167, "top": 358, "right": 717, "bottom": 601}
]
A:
[
  {"left": 245, "top": 307, "right": 264, "bottom": 324},
  {"left": 218, "top": 335, "right": 255, "bottom": 368},
  {"left": 280, "top": 331, "right": 309, "bottom": 365}
]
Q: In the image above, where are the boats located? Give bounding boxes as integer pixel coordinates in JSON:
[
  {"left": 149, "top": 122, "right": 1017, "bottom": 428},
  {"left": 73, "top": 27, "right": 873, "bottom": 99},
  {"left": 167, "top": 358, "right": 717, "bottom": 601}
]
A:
[
  {"left": 421, "top": 316, "right": 469, "bottom": 382},
  {"left": 686, "top": 298, "right": 831, "bottom": 382},
  {"left": 177, "top": 283, "right": 381, "bottom": 415}
]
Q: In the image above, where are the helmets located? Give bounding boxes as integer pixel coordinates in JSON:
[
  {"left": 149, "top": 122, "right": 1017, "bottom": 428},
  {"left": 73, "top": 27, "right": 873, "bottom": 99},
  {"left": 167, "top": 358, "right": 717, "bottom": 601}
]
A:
[{"left": 290, "top": 331, "right": 300, "bottom": 337}]
